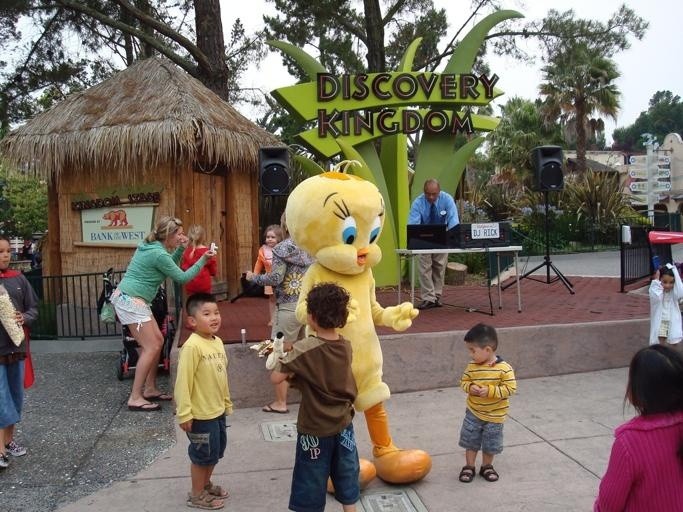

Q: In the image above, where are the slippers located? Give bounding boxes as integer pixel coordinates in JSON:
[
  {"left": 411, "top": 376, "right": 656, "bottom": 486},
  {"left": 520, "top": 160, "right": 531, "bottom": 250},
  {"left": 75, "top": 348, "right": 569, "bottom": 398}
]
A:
[
  {"left": 262, "top": 403, "right": 290, "bottom": 413},
  {"left": 127, "top": 399, "right": 162, "bottom": 411},
  {"left": 144, "top": 393, "right": 173, "bottom": 401}
]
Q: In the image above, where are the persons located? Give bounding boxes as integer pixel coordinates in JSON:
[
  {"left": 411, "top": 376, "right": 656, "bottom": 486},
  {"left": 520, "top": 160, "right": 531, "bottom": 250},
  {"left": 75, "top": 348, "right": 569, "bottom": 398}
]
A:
[
  {"left": 253, "top": 225, "right": 285, "bottom": 327},
  {"left": 1, "top": 235, "right": 41, "bottom": 468},
  {"left": 246, "top": 211, "right": 315, "bottom": 415},
  {"left": 109, "top": 217, "right": 217, "bottom": 411},
  {"left": 179, "top": 223, "right": 217, "bottom": 298},
  {"left": 406, "top": 178, "right": 460, "bottom": 310},
  {"left": 173, "top": 292, "right": 234, "bottom": 510},
  {"left": 648, "top": 262, "right": 683, "bottom": 352},
  {"left": 594, "top": 345, "right": 683, "bottom": 512},
  {"left": 269, "top": 281, "right": 360, "bottom": 512},
  {"left": 21, "top": 239, "right": 36, "bottom": 253},
  {"left": 458, "top": 323, "right": 517, "bottom": 481}
]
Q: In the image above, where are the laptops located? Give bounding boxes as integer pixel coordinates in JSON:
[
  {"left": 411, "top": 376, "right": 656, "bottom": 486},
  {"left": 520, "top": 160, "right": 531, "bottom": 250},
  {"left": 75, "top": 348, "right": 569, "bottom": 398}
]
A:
[{"left": 407, "top": 224, "right": 446, "bottom": 249}]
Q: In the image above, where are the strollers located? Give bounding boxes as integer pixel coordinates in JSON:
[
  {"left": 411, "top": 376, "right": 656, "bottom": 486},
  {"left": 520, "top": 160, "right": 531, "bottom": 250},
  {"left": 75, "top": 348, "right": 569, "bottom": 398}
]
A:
[{"left": 97, "top": 267, "right": 174, "bottom": 382}]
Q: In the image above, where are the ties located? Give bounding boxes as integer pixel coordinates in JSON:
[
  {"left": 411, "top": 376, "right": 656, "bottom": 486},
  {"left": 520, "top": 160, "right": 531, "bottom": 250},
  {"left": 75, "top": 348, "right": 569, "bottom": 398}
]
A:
[{"left": 430, "top": 203, "right": 436, "bottom": 223}]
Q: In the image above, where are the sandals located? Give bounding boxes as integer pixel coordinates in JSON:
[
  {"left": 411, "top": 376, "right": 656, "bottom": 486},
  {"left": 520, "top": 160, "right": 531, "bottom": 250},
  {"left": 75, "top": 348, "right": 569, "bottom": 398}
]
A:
[
  {"left": 205, "top": 481, "right": 232, "bottom": 499},
  {"left": 459, "top": 464, "right": 479, "bottom": 482},
  {"left": 186, "top": 490, "right": 226, "bottom": 509},
  {"left": 481, "top": 464, "right": 501, "bottom": 483}
]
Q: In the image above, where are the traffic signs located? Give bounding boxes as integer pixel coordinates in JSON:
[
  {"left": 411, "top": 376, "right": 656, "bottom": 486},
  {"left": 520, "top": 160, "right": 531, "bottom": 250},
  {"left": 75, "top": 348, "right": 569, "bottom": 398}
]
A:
[
  {"left": 629, "top": 183, "right": 649, "bottom": 193},
  {"left": 631, "top": 196, "right": 649, "bottom": 205},
  {"left": 654, "top": 155, "right": 670, "bottom": 165},
  {"left": 653, "top": 169, "right": 671, "bottom": 179},
  {"left": 629, "top": 169, "right": 647, "bottom": 179},
  {"left": 629, "top": 156, "right": 648, "bottom": 166},
  {"left": 652, "top": 182, "right": 671, "bottom": 191}
]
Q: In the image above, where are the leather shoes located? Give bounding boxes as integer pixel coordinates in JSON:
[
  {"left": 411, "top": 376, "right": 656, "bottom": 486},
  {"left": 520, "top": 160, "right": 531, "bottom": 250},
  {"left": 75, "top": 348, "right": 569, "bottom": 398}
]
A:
[
  {"left": 418, "top": 300, "right": 436, "bottom": 309},
  {"left": 435, "top": 296, "right": 444, "bottom": 307}
]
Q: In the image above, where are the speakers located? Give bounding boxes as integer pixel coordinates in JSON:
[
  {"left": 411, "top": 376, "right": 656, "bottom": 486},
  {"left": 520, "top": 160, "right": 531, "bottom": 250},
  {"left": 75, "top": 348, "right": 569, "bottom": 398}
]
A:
[
  {"left": 259, "top": 145, "right": 290, "bottom": 196},
  {"left": 530, "top": 144, "right": 564, "bottom": 192}
]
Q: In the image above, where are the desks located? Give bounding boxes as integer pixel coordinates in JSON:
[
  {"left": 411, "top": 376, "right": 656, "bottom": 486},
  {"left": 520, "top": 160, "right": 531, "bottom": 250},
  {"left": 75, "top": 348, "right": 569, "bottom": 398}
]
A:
[{"left": 395, "top": 246, "right": 524, "bottom": 312}]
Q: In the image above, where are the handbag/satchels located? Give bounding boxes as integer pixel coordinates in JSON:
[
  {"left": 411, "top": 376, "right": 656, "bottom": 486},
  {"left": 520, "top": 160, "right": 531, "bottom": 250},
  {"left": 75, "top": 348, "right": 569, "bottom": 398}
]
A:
[{"left": 99, "top": 296, "right": 117, "bottom": 325}]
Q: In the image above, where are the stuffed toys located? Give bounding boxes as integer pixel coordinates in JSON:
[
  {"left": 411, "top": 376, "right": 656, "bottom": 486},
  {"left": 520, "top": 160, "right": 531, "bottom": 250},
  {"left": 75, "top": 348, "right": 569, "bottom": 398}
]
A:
[{"left": 285, "top": 160, "right": 431, "bottom": 494}]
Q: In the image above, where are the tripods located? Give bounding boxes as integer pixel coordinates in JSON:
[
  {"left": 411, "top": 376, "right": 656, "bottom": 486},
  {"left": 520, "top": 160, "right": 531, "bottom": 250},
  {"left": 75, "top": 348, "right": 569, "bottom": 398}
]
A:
[{"left": 502, "top": 192, "right": 576, "bottom": 295}]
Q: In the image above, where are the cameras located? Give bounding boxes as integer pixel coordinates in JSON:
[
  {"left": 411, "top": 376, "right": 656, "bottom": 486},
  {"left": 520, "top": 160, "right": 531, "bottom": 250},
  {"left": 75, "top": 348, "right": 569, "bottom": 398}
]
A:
[{"left": 209, "top": 243, "right": 219, "bottom": 252}]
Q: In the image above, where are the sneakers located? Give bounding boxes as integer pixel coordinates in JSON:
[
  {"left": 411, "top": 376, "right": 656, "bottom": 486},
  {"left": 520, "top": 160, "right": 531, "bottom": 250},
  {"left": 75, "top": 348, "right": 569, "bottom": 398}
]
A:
[
  {"left": 5, "top": 441, "right": 26, "bottom": 457},
  {"left": 0, "top": 453, "right": 11, "bottom": 471}
]
